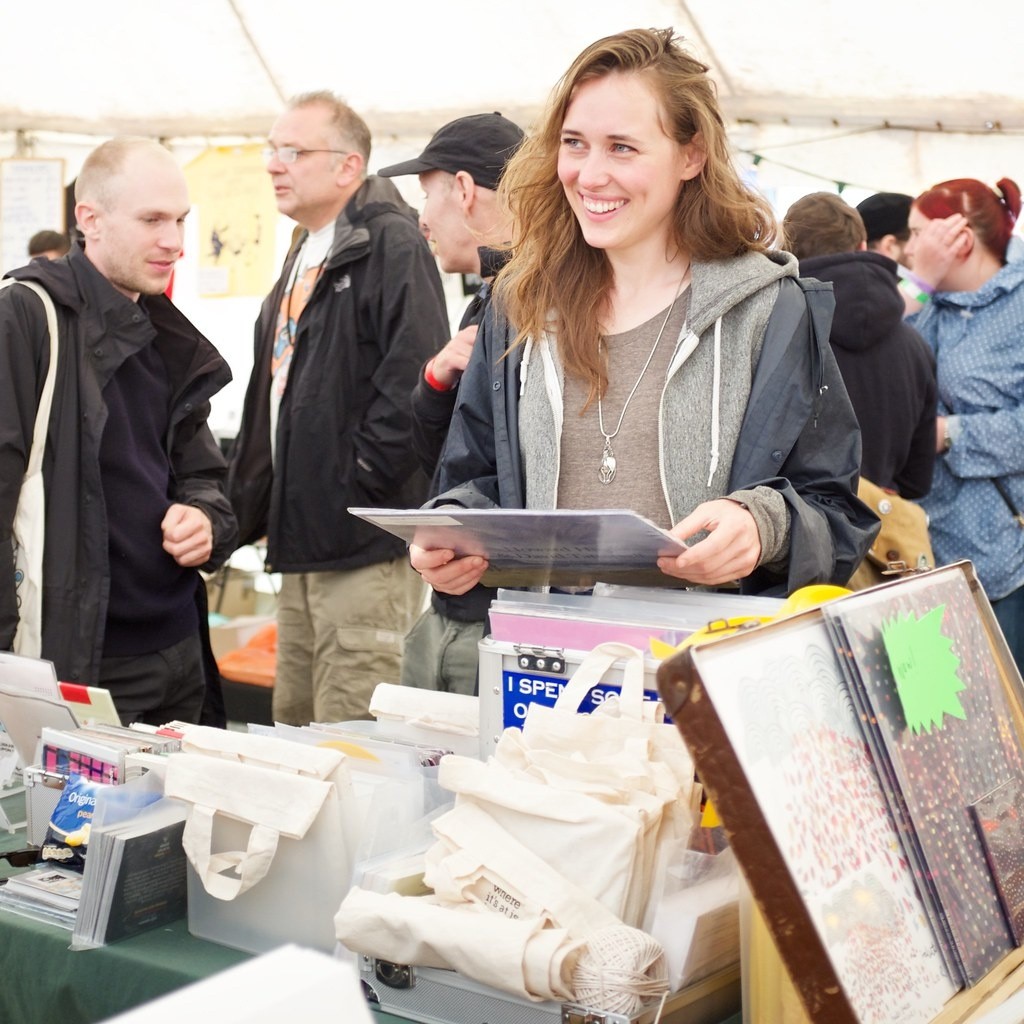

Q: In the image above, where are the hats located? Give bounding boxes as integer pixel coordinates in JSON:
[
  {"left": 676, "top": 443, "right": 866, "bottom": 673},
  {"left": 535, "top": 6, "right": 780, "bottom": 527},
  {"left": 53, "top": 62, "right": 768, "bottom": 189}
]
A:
[{"left": 377, "top": 112, "right": 530, "bottom": 191}]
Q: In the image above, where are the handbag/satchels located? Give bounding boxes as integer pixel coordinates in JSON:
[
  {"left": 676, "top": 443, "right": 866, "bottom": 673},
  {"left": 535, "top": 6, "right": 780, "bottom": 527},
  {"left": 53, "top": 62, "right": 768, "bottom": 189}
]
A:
[
  {"left": 845, "top": 474, "right": 935, "bottom": 593},
  {"left": 0, "top": 277, "right": 58, "bottom": 661}
]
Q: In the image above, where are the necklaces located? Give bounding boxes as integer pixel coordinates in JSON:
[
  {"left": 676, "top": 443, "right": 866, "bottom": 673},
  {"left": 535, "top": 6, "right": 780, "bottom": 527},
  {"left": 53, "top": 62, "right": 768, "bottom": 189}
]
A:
[
  {"left": 595, "top": 258, "right": 691, "bottom": 485},
  {"left": 285, "top": 245, "right": 334, "bottom": 347}
]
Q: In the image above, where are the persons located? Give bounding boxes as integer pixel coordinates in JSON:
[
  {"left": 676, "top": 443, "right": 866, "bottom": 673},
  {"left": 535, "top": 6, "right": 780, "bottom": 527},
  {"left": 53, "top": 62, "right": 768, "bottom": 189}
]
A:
[
  {"left": 376, "top": 110, "right": 544, "bottom": 696},
  {"left": 903, "top": 177, "right": 1024, "bottom": 678},
  {"left": 0, "top": 134, "right": 239, "bottom": 727},
  {"left": 407, "top": 25, "right": 884, "bottom": 696},
  {"left": 855, "top": 192, "right": 920, "bottom": 269},
  {"left": 779, "top": 190, "right": 939, "bottom": 591},
  {"left": 220, "top": 89, "right": 453, "bottom": 727},
  {"left": 25, "top": 230, "right": 71, "bottom": 263}
]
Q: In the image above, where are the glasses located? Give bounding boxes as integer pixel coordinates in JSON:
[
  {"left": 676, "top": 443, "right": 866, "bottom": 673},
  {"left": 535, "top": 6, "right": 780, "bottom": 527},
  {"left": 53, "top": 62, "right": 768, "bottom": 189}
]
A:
[{"left": 264, "top": 148, "right": 347, "bottom": 163}]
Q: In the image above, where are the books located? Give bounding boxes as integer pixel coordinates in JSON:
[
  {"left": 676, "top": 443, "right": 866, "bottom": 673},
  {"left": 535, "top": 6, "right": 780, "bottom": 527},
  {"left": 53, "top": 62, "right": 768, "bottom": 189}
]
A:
[{"left": 5, "top": 718, "right": 199, "bottom": 953}]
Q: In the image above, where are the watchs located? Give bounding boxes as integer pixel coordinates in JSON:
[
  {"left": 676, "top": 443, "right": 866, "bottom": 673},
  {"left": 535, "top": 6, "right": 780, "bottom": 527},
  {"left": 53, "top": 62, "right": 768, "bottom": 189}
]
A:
[{"left": 942, "top": 415, "right": 952, "bottom": 450}]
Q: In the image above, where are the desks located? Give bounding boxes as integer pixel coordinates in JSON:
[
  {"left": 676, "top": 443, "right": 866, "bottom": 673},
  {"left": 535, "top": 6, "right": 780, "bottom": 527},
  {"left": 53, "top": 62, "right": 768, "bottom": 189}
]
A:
[{"left": 1, "top": 867, "right": 422, "bottom": 1024}]
